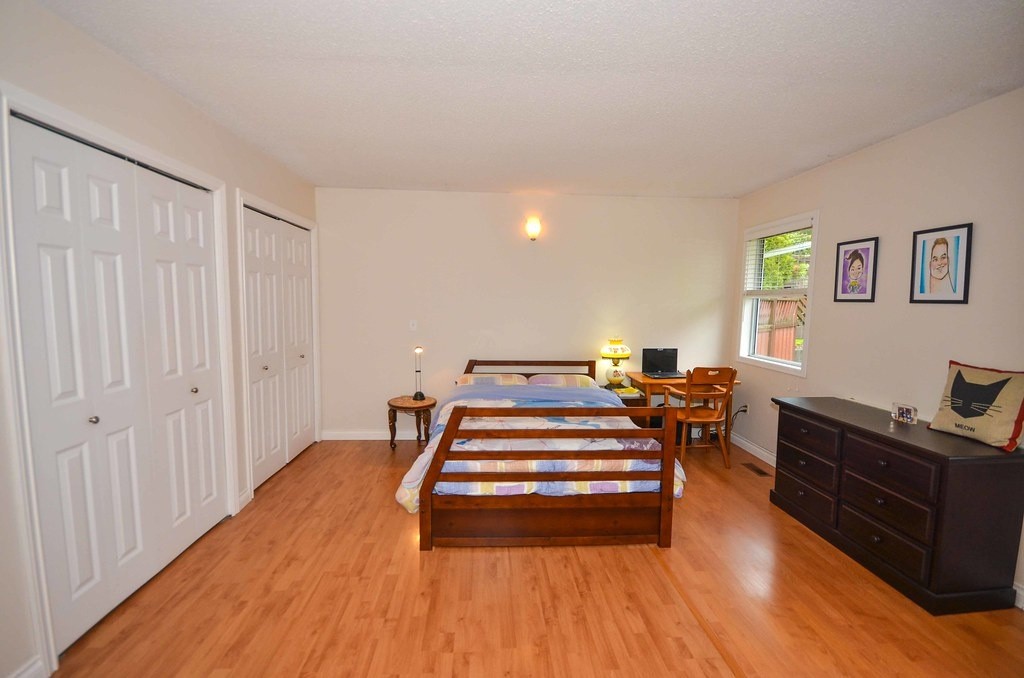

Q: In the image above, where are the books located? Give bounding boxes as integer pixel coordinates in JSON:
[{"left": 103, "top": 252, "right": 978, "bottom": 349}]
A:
[{"left": 612, "top": 386, "right": 641, "bottom": 400}]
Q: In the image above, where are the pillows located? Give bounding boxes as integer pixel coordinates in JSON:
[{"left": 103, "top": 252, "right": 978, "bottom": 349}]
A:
[
  {"left": 925, "top": 360, "right": 1024, "bottom": 452},
  {"left": 459, "top": 373, "right": 528, "bottom": 385},
  {"left": 528, "top": 374, "right": 599, "bottom": 389}
]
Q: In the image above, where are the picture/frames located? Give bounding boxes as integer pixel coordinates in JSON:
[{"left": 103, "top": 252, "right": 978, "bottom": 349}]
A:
[
  {"left": 909, "top": 223, "right": 973, "bottom": 304},
  {"left": 833, "top": 236, "right": 879, "bottom": 303}
]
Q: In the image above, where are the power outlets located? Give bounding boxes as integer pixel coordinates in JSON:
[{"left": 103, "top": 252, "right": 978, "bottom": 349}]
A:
[{"left": 743, "top": 403, "right": 749, "bottom": 414}]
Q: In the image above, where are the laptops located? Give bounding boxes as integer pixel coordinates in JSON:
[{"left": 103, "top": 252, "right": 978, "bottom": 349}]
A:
[{"left": 643, "top": 348, "right": 686, "bottom": 379}]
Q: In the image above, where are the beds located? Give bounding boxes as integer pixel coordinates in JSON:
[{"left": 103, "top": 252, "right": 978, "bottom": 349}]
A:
[{"left": 394, "top": 360, "right": 686, "bottom": 547}]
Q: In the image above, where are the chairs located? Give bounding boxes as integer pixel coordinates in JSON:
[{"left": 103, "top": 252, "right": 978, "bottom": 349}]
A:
[{"left": 662, "top": 366, "right": 736, "bottom": 468}]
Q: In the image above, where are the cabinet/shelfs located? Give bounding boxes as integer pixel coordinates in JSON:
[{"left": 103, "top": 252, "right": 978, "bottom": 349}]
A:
[
  {"left": 213, "top": 171, "right": 324, "bottom": 516},
  {"left": 769, "top": 397, "right": 1024, "bottom": 616},
  {"left": 0, "top": 80, "right": 227, "bottom": 672}
]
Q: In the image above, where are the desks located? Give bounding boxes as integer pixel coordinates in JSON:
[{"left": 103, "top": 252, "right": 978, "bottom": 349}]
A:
[
  {"left": 387, "top": 395, "right": 437, "bottom": 452},
  {"left": 624, "top": 371, "right": 742, "bottom": 454}
]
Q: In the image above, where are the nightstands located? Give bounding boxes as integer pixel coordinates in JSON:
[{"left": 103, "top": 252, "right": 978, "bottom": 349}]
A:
[{"left": 599, "top": 386, "right": 647, "bottom": 428}]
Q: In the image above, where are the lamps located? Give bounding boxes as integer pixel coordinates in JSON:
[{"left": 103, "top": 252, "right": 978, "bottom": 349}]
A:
[
  {"left": 599, "top": 337, "right": 633, "bottom": 389},
  {"left": 525, "top": 216, "right": 542, "bottom": 241},
  {"left": 413, "top": 346, "right": 425, "bottom": 401}
]
